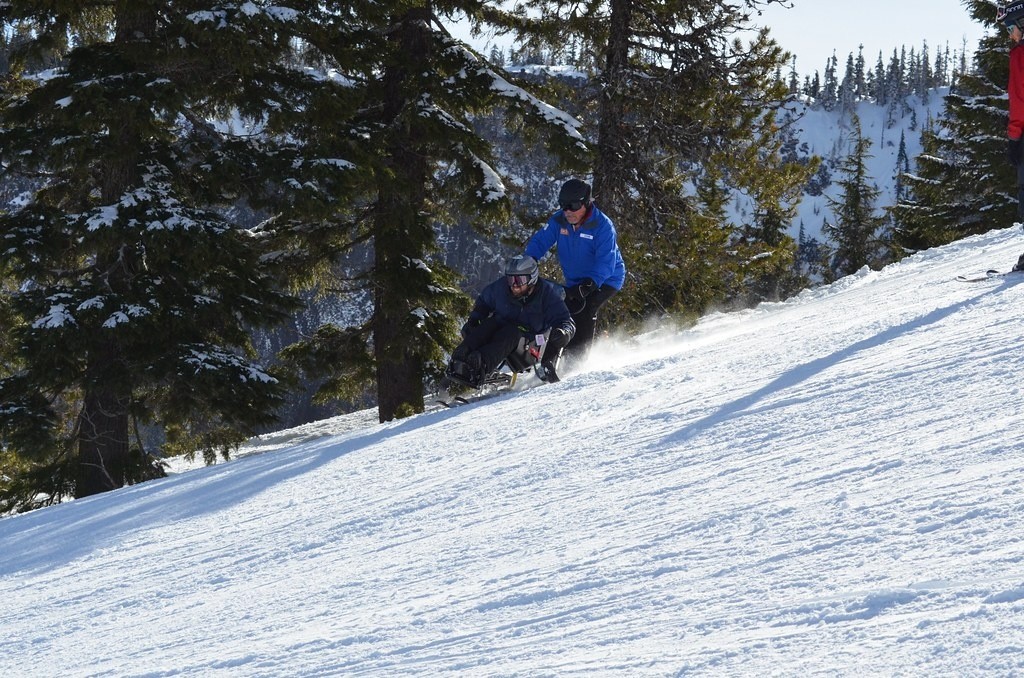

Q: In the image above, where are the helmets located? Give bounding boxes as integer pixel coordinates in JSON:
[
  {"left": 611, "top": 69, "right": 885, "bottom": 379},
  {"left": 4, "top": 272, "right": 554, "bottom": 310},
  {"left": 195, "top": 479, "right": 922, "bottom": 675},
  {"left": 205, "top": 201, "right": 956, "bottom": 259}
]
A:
[
  {"left": 557, "top": 179, "right": 591, "bottom": 208},
  {"left": 505, "top": 254, "right": 539, "bottom": 285}
]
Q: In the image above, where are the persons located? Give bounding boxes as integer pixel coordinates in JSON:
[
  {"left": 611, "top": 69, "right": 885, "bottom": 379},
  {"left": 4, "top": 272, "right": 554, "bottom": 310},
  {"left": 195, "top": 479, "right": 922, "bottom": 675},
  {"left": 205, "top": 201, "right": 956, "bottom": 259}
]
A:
[
  {"left": 451, "top": 254, "right": 576, "bottom": 383},
  {"left": 521, "top": 179, "right": 625, "bottom": 367},
  {"left": 995, "top": 0, "right": 1024, "bottom": 271}
]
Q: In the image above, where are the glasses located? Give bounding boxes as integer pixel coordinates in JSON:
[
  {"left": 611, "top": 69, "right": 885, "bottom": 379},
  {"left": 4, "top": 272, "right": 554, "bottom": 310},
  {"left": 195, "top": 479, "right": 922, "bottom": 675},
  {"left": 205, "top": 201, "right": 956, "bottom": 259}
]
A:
[
  {"left": 559, "top": 201, "right": 582, "bottom": 212},
  {"left": 507, "top": 275, "right": 531, "bottom": 287}
]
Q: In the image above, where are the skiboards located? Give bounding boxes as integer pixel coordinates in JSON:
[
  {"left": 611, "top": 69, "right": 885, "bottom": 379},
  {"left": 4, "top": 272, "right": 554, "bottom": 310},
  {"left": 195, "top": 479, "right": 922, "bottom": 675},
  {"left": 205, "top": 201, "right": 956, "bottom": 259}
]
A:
[{"left": 954, "top": 259, "right": 1024, "bottom": 284}]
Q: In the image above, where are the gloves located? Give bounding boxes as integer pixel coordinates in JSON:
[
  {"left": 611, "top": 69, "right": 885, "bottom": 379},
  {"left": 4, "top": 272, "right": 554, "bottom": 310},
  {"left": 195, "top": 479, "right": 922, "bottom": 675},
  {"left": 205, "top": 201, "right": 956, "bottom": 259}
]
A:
[
  {"left": 565, "top": 279, "right": 597, "bottom": 305},
  {"left": 547, "top": 327, "right": 574, "bottom": 349},
  {"left": 461, "top": 318, "right": 479, "bottom": 338}
]
